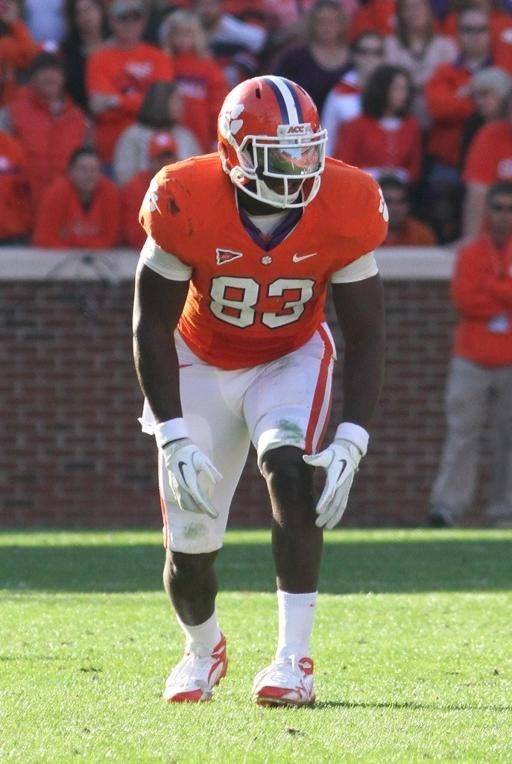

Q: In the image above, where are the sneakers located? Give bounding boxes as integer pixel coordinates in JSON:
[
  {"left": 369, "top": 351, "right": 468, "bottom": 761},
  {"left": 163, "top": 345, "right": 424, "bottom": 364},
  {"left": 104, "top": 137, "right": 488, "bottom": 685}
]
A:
[
  {"left": 163, "top": 631, "right": 231, "bottom": 705},
  {"left": 253, "top": 656, "right": 318, "bottom": 707},
  {"left": 429, "top": 505, "right": 510, "bottom": 532}
]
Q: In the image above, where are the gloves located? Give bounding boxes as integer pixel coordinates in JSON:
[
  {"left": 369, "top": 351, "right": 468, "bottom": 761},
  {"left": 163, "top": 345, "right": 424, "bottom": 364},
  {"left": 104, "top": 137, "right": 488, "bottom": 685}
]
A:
[
  {"left": 154, "top": 417, "right": 223, "bottom": 521},
  {"left": 302, "top": 423, "right": 370, "bottom": 530}
]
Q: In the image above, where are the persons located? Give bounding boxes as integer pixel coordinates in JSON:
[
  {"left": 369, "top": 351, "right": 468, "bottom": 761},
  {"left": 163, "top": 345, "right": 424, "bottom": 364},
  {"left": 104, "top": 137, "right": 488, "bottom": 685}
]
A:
[
  {"left": 129, "top": 73, "right": 390, "bottom": 708},
  {"left": 1, "top": 1, "right": 511, "bottom": 249},
  {"left": 421, "top": 175, "right": 511, "bottom": 530}
]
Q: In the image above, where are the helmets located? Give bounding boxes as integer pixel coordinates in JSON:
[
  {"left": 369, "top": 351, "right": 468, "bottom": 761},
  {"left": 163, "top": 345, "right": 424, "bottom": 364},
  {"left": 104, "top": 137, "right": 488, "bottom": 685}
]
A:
[{"left": 217, "top": 75, "right": 326, "bottom": 208}]
